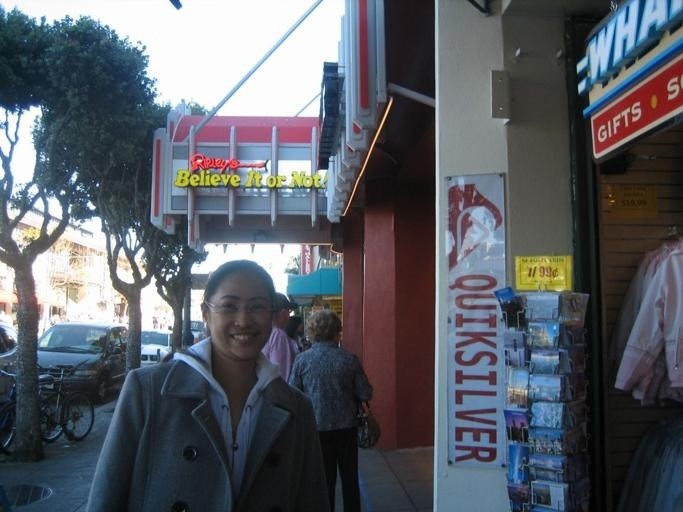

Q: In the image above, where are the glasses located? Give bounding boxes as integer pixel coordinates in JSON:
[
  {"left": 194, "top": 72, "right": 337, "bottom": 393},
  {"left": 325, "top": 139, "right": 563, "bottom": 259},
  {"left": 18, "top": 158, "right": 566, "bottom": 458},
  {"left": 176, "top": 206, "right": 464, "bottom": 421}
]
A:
[{"left": 205, "top": 304, "right": 272, "bottom": 312}]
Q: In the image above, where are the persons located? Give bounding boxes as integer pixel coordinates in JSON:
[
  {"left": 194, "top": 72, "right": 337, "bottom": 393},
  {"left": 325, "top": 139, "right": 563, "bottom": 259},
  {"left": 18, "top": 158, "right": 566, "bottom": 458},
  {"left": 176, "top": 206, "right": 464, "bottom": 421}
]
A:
[
  {"left": 286, "top": 316, "right": 303, "bottom": 354},
  {"left": 288, "top": 309, "right": 372, "bottom": 511},
  {"left": 261, "top": 292, "right": 295, "bottom": 383},
  {"left": 84, "top": 259, "right": 331, "bottom": 512},
  {"left": 151, "top": 310, "right": 159, "bottom": 329}
]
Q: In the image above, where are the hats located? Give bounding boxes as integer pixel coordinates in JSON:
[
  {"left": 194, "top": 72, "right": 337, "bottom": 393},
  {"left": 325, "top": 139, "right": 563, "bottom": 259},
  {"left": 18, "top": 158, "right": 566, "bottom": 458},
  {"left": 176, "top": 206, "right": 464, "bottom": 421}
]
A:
[{"left": 275, "top": 293, "right": 298, "bottom": 311}]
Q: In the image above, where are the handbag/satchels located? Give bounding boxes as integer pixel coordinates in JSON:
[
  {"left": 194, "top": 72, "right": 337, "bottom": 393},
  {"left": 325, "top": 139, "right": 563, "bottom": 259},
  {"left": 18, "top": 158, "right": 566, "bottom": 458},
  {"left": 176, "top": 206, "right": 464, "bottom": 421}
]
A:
[{"left": 355, "top": 401, "right": 379, "bottom": 449}]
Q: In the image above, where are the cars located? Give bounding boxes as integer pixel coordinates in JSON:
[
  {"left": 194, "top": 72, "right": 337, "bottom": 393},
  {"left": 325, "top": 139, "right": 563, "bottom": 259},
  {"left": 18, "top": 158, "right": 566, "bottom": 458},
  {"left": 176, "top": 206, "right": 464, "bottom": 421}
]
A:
[
  {"left": 0, "top": 323, "right": 19, "bottom": 401},
  {"left": 37, "top": 323, "right": 129, "bottom": 402},
  {"left": 140, "top": 330, "right": 173, "bottom": 366},
  {"left": 167, "top": 320, "right": 205, "bottom": 344}
]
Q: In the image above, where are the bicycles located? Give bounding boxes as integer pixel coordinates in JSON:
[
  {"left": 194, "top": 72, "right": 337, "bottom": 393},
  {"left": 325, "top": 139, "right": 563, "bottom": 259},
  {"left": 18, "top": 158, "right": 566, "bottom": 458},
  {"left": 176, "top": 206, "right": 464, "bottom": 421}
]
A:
[
  {"left": 0, "top": 370, "right": 70, "bottom": 443},
  {"left": 0, "top": 364, "right": 95, "bottom": 452}
]
